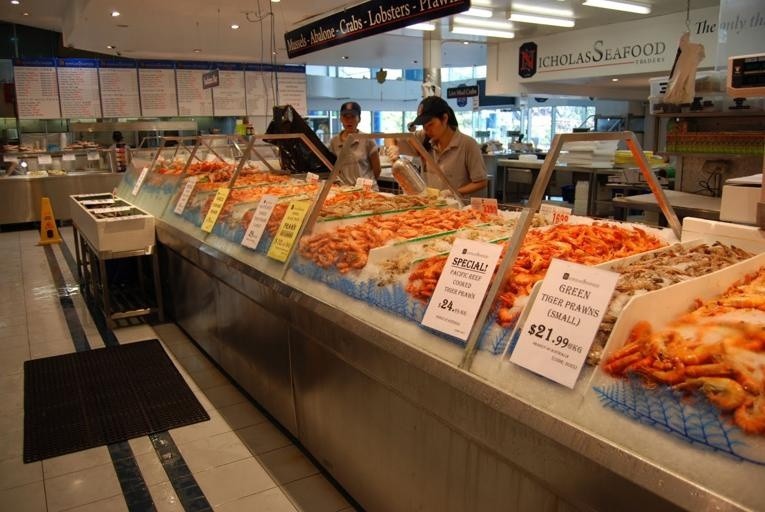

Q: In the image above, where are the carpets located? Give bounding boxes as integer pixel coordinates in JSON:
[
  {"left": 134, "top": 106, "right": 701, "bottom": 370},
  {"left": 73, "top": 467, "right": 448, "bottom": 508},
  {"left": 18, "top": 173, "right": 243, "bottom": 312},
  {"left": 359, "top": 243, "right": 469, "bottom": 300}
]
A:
[{"left": 23, "top": 338, "right": 210, "bottom": 463}]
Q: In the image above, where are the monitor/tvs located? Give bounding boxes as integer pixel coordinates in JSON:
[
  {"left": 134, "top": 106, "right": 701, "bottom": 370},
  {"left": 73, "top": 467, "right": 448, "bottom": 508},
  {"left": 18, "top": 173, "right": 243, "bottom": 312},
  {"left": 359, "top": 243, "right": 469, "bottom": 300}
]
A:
[{"left": 476, "top": 79, "right": 520, "bottom": 110}]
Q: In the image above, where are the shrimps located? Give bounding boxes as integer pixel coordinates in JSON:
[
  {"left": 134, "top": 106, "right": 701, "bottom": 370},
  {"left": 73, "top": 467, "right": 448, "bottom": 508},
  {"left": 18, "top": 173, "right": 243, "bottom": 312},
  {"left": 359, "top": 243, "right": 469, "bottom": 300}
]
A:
[{"left": 152, "top": 158, "right": 765, "bottom": 438}]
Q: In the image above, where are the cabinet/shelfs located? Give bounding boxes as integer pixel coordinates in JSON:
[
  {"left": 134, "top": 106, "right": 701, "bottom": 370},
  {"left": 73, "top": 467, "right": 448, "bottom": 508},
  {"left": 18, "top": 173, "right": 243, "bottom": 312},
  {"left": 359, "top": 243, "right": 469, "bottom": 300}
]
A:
[{"left": 72, "top": 221, "right": 165, "bottom": 329}]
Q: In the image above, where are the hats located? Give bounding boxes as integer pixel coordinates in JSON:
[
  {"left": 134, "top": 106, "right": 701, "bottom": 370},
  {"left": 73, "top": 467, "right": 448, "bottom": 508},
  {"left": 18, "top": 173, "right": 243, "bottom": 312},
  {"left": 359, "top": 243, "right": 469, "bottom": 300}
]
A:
[
  {"left": 340, "top": 101, "right": 360, "bottom": 116},
  {"left": 412, "top": 97, "right": 448, "bottom": 125}
]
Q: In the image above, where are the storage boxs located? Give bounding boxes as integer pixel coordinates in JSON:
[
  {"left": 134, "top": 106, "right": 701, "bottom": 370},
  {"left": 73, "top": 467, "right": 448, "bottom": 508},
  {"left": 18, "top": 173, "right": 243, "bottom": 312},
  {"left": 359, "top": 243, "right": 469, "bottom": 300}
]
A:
[
  {"left": 647, "top": 71, "right": 724, "bottom": 115},
  {"left": 680, "top": 217, "right": 765, "bottom": 253},
  {"left": 719, "top": 172, "right": 762, "bottom": 226}
]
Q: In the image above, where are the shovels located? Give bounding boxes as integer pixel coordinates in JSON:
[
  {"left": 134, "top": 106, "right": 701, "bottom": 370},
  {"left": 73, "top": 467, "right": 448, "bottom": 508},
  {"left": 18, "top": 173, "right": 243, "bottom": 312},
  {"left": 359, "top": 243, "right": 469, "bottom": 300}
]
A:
[{"left": 383, "top": 146, "right": 428, "bottom": 196}]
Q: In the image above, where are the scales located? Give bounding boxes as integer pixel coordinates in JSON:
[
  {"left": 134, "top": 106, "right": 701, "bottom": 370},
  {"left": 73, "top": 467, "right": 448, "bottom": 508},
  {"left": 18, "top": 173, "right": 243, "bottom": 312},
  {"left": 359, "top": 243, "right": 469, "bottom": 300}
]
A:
[
  {"left": 507, "top": 131, "right": 533, "bottom": 151},
  {"left": 474, "top": 131, "right": 501, "bottom": 151}
]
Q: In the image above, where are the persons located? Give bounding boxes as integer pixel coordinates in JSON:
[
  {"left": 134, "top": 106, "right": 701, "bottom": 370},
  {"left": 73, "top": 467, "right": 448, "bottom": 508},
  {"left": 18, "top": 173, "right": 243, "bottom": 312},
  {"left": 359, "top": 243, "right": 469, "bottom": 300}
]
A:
[
  {"left": 164, "top": 131, "right": 179, "bottom": 147},
  {"left": 0, "top": 137, "right": 20, "bottom": 177},
  {"left": 385, "top": 94, "right": 489, "bottom": 199},
  {"left": 329, "top": 101, "right": 381, "bottom": 191},
  {"left": 104, "top": 130, "right": 130, "bottom": 172}
]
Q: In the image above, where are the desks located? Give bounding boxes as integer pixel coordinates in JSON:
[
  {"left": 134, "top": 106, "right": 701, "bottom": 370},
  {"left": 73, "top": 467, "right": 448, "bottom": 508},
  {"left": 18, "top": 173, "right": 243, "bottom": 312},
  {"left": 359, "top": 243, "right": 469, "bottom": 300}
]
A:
[
  {"left": 497, "top": 159, "right": 666, "bottom": 217},
  {"left": 612, "top": 189, "right": 721, "bottom": 229}
]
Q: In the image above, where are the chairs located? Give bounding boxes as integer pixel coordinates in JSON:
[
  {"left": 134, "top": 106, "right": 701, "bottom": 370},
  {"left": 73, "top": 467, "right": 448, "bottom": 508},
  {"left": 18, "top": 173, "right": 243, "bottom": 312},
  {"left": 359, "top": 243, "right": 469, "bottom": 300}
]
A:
[{"left": 506, "top": 168, "right": 534, "bottom": 203}]
